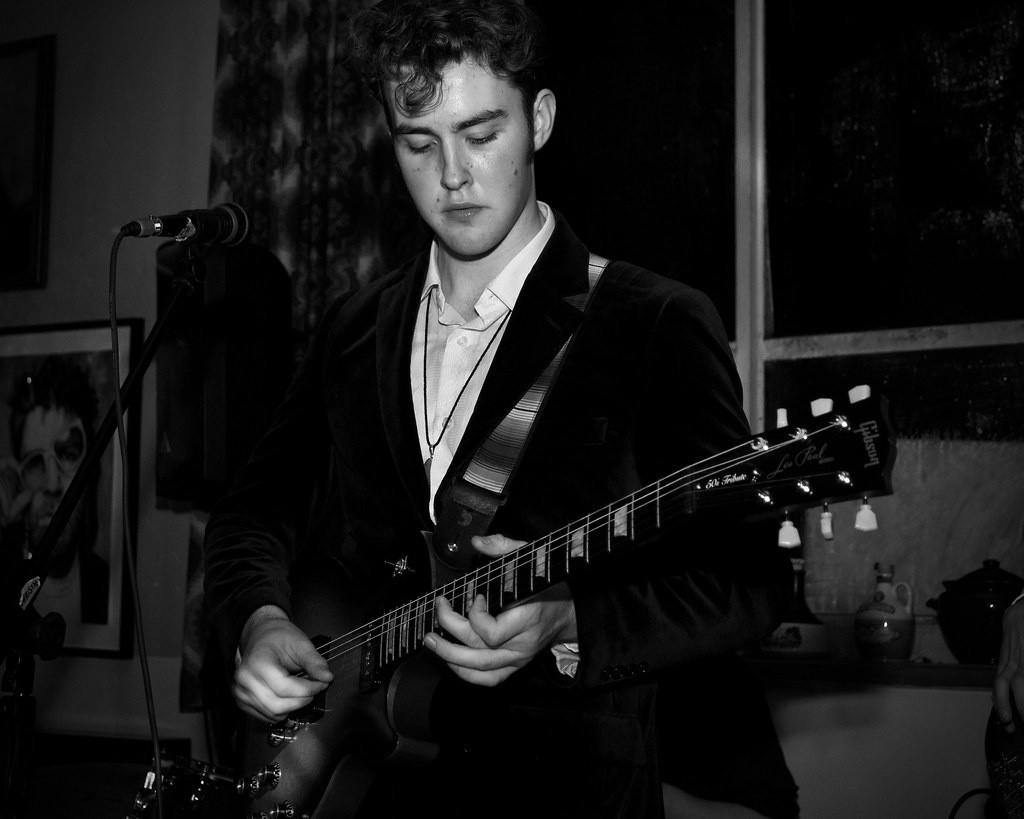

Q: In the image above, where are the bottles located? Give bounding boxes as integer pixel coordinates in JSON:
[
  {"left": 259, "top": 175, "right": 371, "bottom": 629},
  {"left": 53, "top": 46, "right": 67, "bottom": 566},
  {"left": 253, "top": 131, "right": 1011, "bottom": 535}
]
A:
[
  {"left": 924, "top": 559, "right": 1024, "bottom": 667},
  {"left": 856, "top": 561, "right": 915, "bottom": 664},
  {"left": 758, "top": 569, "right": 826, "bottom": 663}
]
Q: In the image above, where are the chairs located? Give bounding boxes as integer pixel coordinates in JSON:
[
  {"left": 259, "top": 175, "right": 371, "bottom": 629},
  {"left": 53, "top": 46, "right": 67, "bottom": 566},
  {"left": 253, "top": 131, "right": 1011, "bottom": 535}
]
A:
[{"left": 801, "top": 438, "right": 1024, "bottom": 663}]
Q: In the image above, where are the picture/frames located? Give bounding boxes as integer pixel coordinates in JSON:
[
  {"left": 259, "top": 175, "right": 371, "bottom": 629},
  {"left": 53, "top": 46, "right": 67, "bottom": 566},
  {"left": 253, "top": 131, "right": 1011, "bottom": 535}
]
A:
[
  {"left": 0, "top": 317, "right": 145, "bottom": 660},
  {"left": 0, "top": 36, "right": 57, "bottom": 292}
]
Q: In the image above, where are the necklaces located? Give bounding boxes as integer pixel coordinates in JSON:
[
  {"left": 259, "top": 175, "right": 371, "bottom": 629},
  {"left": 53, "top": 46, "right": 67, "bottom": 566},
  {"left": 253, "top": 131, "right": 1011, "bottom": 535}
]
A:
[{"left": 424, "top": 290, "right": 511, "bottom": 482}]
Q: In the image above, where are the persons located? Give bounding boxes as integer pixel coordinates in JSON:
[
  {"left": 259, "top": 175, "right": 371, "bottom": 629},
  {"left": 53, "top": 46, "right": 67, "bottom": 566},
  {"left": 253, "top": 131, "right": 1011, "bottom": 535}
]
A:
[
  {"left": 9, "top": 358, "right": 111, "bottom": 625},
  {"left": 992, "top": 592, "right": 1024, "bottom": 733},
  {"left": 200, "top": 0, "right": 803, "bottom": 819}
]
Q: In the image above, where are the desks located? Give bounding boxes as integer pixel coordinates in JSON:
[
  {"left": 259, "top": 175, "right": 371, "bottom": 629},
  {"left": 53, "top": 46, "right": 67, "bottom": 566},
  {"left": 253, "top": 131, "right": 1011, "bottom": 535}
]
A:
[{"left": 663, "top": 612, "right": 996, "bottom": 819}]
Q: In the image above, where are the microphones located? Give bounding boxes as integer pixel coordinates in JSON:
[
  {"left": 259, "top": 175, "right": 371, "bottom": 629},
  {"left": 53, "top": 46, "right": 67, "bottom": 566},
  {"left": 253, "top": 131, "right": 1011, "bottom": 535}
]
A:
[{"left": 123, "top": 201, "right": 248, "bottom": 247}]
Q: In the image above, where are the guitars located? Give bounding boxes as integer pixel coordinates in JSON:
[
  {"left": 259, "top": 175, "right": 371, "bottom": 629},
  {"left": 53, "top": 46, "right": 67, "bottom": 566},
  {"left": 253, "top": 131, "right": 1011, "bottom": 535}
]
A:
[{"left": 201, "top": 383, "right": 896, "bottom": 819}]
